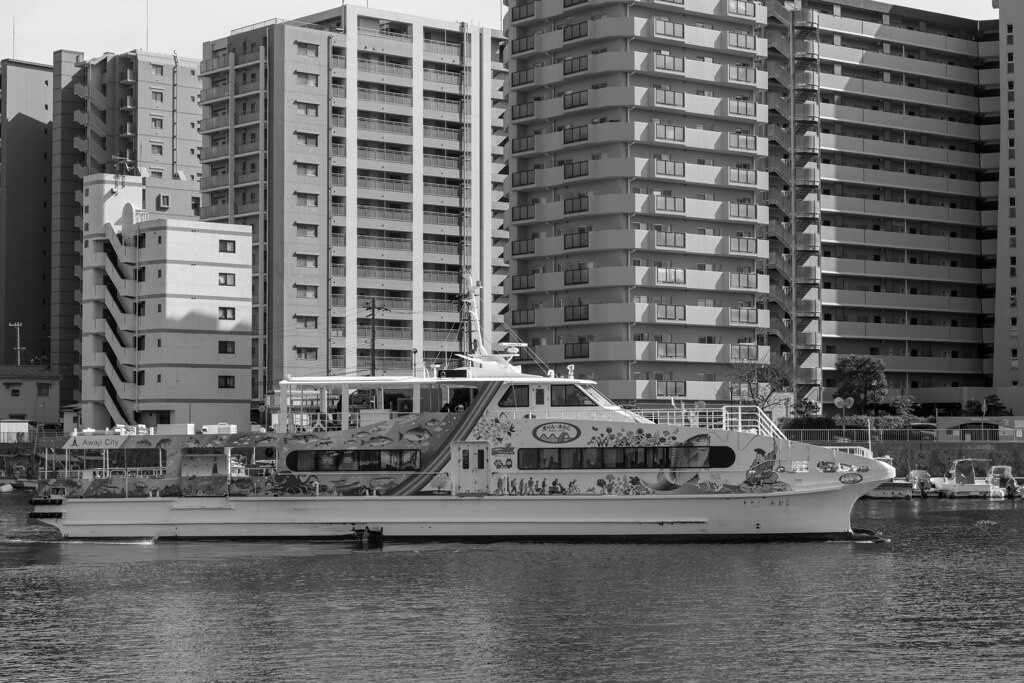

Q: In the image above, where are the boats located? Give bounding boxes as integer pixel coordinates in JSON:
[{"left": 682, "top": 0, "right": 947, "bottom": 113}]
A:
[
  {"left": 934, "top": 456, "right": 1005, "bottom": 501},
  {"left": 861, "top": 471, "right": 915, "bottom": 502},
  {"left": 30, "top": 277, "right": 902, "bottom": 551}
]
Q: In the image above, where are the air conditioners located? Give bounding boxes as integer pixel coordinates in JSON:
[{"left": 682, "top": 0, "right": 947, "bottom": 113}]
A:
[{"left": 398, "top": 398, "right": 413, "bottom": 412}]
